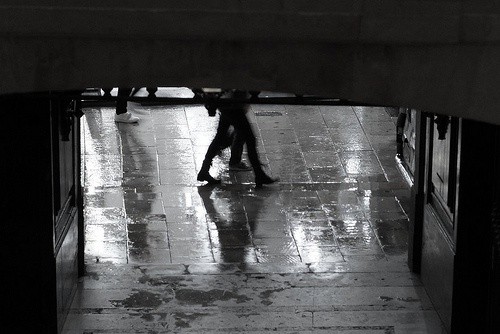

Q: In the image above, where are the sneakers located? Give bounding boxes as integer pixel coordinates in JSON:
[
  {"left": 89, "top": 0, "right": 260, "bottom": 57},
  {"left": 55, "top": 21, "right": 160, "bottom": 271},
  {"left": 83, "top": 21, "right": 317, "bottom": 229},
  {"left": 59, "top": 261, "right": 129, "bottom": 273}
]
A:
[
  {"left": 114, "top": 112, "right": 139, "bottom": 123},
  {"left": 227, "top": 162, "right": 252, "bottom": 171}
]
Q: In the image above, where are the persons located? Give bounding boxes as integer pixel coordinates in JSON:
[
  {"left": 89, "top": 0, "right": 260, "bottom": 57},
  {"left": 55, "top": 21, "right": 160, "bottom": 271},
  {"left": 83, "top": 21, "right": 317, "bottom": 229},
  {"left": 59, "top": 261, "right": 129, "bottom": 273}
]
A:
[
  {"left": 194, "top": 90, "right": 279, "bottom": 190},
  {"left": 114, "top": 86, "right": 137, "bottom": 124},
  {"left": 212, "top": 125, "right": 251, "bottom": 171}
]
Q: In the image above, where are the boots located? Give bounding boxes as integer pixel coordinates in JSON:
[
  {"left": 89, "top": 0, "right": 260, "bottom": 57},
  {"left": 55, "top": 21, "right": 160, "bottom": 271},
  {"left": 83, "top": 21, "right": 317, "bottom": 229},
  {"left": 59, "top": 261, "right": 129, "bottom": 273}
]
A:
[
  {"left": 252, "top": 166, "right": 279, "bottom": 188},
  {"left": 198, "top": 161, "right": 220, "bottom": 185}
]
